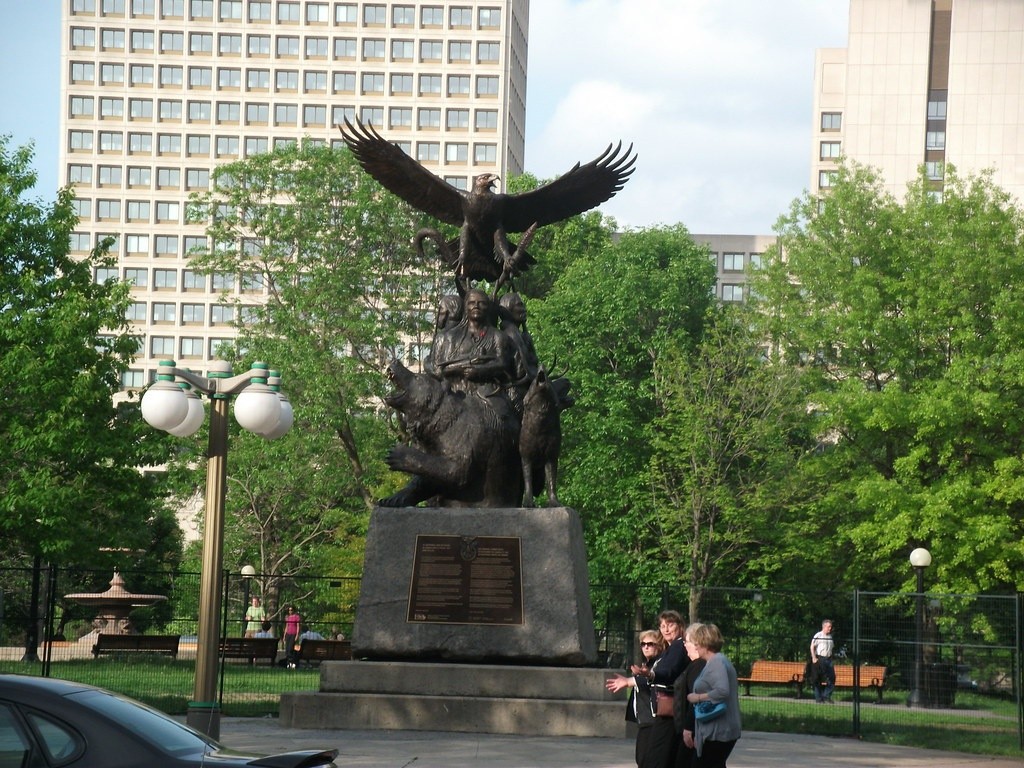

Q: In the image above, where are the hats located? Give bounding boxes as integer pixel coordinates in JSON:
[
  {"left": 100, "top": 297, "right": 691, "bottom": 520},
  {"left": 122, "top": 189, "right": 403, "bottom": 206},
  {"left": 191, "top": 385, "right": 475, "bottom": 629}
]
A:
[{"left": 812, "top": 655, "right": 825, "bottom": 669}]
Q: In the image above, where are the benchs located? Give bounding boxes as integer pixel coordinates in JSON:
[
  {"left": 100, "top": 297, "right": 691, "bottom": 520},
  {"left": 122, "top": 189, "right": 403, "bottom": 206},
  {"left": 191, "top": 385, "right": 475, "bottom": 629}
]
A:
[
  {"left": 736, "top": 660, "right": 809, "bottom": 699},
  {"left": 292, "top": 638, "right": 350, "bottom": 668},
  {"left": 92, "top": 632, "right": 181, "bottom": 660},
  {"left": 809, "top": 664, "right": 887, "bottom": 703},
  {"left": 219, "top": 637, "right": 280, "bottom": 666}
]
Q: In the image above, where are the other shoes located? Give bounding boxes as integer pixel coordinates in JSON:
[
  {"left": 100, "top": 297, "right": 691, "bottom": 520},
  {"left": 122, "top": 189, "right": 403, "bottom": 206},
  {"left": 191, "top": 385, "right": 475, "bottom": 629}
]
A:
[
  {"left": 816, "top": 699, "right": 826, "bottom": 704},
  {"left": 286, "top": 662, "right": 296, "bottom": 669},
  {"left": 823, "top": 697, "right": 834, "bottom": 704}
]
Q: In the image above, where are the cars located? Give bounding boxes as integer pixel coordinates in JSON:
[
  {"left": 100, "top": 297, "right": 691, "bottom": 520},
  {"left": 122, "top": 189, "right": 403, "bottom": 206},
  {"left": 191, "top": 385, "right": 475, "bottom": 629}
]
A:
[
  {"left": 957, "top": 664, "right": 979, "bottom": 689},
  {"left": 614, "top": 632, "right": 626, "bottom": 642},
  {"left": 0, "top": 672, "right": 339, "bottom": 768},
  {"left": 973, "top": 672, "right": 1017, "bottom": 692}
]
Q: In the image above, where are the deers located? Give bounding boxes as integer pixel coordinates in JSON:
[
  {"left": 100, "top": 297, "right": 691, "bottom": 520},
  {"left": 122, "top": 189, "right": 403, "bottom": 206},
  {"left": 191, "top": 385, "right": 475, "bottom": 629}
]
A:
[{"left": 484, "top": 353, "right": 570, "bottom": 509}]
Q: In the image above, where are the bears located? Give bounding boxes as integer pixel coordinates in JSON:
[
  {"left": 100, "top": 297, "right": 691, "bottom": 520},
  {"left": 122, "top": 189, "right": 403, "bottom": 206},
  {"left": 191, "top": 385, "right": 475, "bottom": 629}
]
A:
[{"left": 377, "top": 358, "right": 521, "bottom": 507}]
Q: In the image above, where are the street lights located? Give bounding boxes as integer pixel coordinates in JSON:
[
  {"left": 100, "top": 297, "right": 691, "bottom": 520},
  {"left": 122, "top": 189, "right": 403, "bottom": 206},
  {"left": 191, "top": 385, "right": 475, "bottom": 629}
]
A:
[
  {"left": 625, "top": 612, "right": 635, "bottom": 644},
  {"left": 905, "top": 549, "right": 932, "bottom": 707},
  {"left": 141, "top": 359, "right": 294, "bottom": 743},
  {"left": 241, "top": 565, "right": 256, "bottom": 637}
]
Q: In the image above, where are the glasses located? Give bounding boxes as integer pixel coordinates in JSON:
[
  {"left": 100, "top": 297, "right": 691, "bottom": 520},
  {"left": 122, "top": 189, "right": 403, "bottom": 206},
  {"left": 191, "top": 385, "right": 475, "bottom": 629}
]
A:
[
  {"left": 682, "top": 640, "right": 696, "bottom": 645},
  {"left": 288, "top": 610, "right": 293, "bottom": 611},
  {"left": 640, "top": 641, "right": 658, "bottom": 647}
]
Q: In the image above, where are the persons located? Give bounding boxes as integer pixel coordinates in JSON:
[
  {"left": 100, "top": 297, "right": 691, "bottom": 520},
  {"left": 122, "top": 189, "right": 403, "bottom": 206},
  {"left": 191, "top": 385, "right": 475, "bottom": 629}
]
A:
[
  {"left": 605, "top": 610, "right": 741, "bottom": 768},
  {"left": 809, "top": 618, "right": 836, "bottom": 705},
  {"left": 422, "top": 289, "right": 543, "bottom": 404},
  {"left": 242, "top": 595, "right": 345, "bottom": 668}
]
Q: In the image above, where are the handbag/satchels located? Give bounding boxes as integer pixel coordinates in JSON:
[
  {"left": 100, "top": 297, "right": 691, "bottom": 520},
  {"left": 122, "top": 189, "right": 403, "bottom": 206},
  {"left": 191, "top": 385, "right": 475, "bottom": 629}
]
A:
[
  {"left": 294, "top": 641, "right": 301, "bottom": 651},
  {"left": 654, "top": 684, "right": 673, "bottom": 716},
  {"left": 693, "top": 702, "right": 728, "bottom": 724}
]
